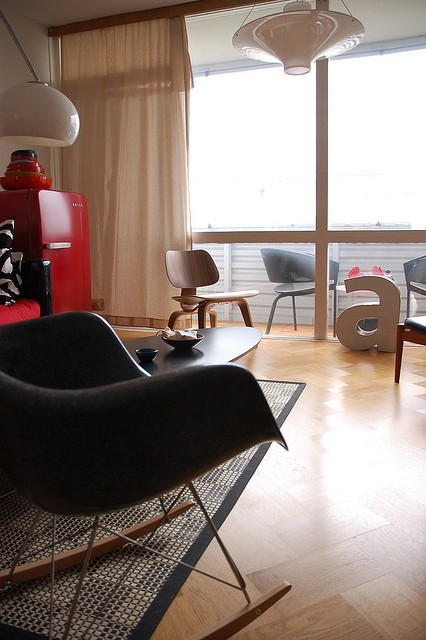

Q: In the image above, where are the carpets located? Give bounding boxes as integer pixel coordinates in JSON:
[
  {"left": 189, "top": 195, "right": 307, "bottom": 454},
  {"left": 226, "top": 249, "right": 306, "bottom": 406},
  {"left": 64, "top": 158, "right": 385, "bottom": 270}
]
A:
[{"left": 1, "top": 382, "right": 305, "bottom": 640}]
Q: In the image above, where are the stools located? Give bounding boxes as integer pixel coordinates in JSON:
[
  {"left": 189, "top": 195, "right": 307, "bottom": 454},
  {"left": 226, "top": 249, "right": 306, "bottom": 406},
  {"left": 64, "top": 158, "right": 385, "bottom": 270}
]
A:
[{"left": 395, "top": 317, "right": 426, "bottom": 383}]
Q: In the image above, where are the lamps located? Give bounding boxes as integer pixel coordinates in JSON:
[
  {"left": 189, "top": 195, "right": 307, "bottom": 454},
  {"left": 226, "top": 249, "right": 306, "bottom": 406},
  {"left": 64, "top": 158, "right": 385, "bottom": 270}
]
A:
[{"left": 232, "top": 1, "right": 365, "bottom": 75}]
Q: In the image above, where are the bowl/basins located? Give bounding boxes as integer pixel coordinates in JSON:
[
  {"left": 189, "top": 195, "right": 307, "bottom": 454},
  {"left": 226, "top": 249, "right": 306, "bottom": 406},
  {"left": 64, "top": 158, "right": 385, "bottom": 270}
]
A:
[{"left": 136, "top": 347, "right": 159, "bottom": 363}]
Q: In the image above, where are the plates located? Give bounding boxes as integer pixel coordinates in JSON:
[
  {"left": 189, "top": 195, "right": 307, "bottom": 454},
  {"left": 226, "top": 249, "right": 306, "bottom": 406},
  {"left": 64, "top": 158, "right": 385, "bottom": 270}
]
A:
[{"left": 161, "top": 333, "right": 204, "bottom": 351}]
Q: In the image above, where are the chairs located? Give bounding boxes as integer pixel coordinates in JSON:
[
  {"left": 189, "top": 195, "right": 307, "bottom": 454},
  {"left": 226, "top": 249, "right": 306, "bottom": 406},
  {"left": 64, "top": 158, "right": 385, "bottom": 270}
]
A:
[
  {"left": 0, "top": 0, "right": 80, "bottom": 148},
  {"left": 165, "top": 250, "right": 261, "bottom": 329},
  {"left": 260, "top": 248, "right": 341, "bottom": 338},
  {"left": 403, "top": 255, "right": 426, "bottom": 317}
]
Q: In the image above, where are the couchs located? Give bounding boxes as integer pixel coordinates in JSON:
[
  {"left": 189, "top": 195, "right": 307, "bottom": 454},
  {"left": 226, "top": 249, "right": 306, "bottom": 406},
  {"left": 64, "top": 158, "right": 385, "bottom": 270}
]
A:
[
  {"left": 0, "top": 311, "right": 292, "bottom": 639},
  {"left": 1, "top": 258, "right": 52, "bottom": 325}
]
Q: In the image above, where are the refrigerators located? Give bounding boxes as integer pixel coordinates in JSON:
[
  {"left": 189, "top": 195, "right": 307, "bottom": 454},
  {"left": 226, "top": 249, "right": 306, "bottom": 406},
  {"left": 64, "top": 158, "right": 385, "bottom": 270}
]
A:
[{"left": 0, "top": 190, "right": 93, "bottom": 314}]
